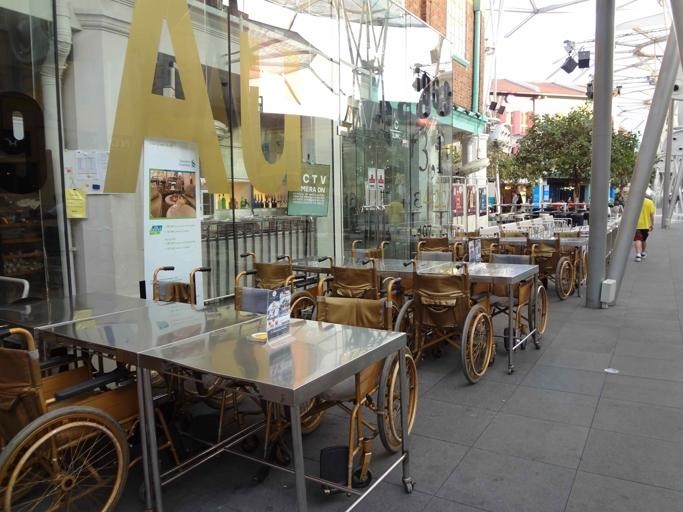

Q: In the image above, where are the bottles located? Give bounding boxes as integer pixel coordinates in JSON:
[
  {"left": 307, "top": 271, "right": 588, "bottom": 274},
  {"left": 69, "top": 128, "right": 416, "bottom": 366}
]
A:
[{"left": 218, "top": 192, "right": 277, "bottom": 209}]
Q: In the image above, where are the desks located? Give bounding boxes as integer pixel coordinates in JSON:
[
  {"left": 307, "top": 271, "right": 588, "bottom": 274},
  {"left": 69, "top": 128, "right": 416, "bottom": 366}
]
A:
[{"left": 2, "top": 238, "right": 589, "bottom": 511}]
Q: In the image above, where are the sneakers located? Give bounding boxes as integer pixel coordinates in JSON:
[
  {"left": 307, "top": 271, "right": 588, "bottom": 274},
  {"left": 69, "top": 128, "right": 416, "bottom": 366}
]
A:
[{"left": 635, "top": 251, "right": 647, "bottom": 262}]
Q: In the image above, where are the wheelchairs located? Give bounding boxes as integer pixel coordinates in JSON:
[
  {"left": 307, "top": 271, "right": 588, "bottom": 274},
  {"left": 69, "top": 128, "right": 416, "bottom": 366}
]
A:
[
  {"left": 0, "top": 322, "right": 197, "bottom": 512},
  {"left": 248, "top": 275, "right": 421, "bottom": 496},
  {"left": 393, "top": 258, "right": 498, "bottom": 385},
  {"left": 138, "top": 222, "right": 588, "bottom": 428}
]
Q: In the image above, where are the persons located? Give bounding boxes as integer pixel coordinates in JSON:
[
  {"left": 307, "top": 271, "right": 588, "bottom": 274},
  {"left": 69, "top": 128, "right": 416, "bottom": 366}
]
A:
[
  {"left": 634, "top": 196, "right": 656, "bottom": 262},
  {"left": 515, "top": 193, "right": 523, "bottom": 211},
  {"left": 510, "top": 191, "right": 517, "bottom": 213},
  {"left": 383, "top": 193, "right": 405, "bottom": 259}
]
